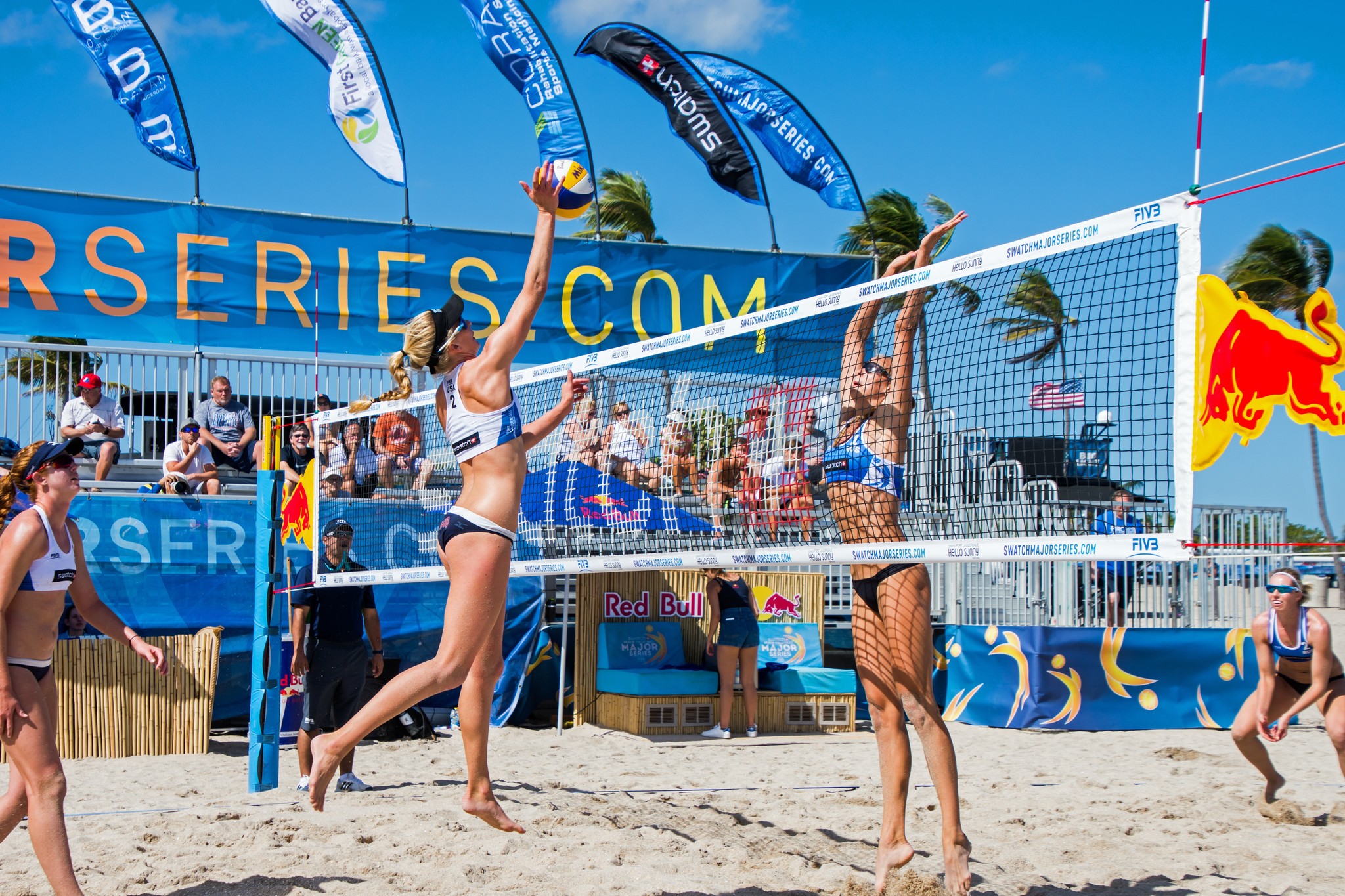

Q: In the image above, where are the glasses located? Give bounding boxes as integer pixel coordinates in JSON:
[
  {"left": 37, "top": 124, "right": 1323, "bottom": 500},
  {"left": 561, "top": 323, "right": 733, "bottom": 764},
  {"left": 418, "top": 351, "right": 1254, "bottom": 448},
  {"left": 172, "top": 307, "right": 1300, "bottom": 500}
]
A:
[
  {"left": 328, "top": 531, "right": 354, "bottom": 540},
  {"left": 182, "top": 427, "right": 199, "bottom": 433},
  {"left": 1265, "top": 584, "right": 1297, "bottom": 593},
  {"left": 758, "top": 410, "right": 770, "bottom": 416},
  {"left": 78, "top": 386, "right": 99, "bottom": 391},
  {"left": 805, "top": 415, "right": 815, "bottom": 419},
  {"left": 317, "top": 401, "right": 329, "bottom": 406},
  {"left": 294, "top": 434, "right": 308, "bottom": 438},
  {"left": 326, "top": 478, "right": 341, "bottom": 483},
  {"left": 589, "top": 412, "right": 597, "bottom": 417},
  {"left": 617, "top": 410, "right": 630, "bottom": 416},
  {"left": 438, "top": 316, "right": 468, "bottom": 353},
  {"left": 25, "top": 453, "right": 74, "bottom": 480},
  {"left": 348, "top": 421, "right": 361, "bottom": 427},
  {"left": 860, "top": 361, "right": 893, "bottom": 379}
]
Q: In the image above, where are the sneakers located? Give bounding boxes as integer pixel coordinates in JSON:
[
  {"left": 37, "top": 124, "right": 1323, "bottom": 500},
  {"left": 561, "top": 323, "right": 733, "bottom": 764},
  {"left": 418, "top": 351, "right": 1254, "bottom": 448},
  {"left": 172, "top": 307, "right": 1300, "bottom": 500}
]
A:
[
  {"left": 745, "top": 724, "right": 758, "bottom": 737},
  {"left": 336, "top": 772, "right": 373, "bottom": 791},
  {"left": 702, "top": 723, "right": 730, "bottom": 739},
  {"left": 296, "top": 775, "right": 310, "bottom": 791}
]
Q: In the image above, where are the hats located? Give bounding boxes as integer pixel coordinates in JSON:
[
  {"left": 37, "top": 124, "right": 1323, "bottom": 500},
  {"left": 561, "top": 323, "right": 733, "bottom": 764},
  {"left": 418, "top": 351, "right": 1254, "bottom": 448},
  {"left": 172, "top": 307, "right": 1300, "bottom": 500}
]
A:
[
  {"left": 24, "top": 437, "right": 84, "bottom": 480},
  {"left": 76, "top": 373, "right": 102, "bottom": 388},
  {"left": 181, "top": 417, "right": 200, "bottom": 431},
  {"left": 323, "top": 467, "right": 344, "bottom": 482},
  {"left": 425, "top": 294, "right": 464, "bottom": 375},
  {"left": 168, "top": 471, "right": 192, "bottom": 493},
  {"left": 313, "top": 393, "right": 330, "bottom": 411},
  {"left": 765, "top": 662, "right": 788, "bottom": 672},
  {"left": 665, "top": 410, "right": 688, "bottom": 422},
  {"left": 323, "top": 518, "right": 354, "bottom": 537},
  {"left": 746, "top": 400, "right": 776, "bottom": 416}
]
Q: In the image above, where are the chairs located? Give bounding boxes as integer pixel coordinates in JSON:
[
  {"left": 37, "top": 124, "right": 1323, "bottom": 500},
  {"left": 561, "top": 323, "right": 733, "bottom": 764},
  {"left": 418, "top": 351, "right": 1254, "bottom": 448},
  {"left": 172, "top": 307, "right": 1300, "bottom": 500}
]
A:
[
  {"left": 597, "top": 621, "right": 718, "bottom": 695},
  {"left": 757, "top": 623, "right": 857, "bottom": 695}
]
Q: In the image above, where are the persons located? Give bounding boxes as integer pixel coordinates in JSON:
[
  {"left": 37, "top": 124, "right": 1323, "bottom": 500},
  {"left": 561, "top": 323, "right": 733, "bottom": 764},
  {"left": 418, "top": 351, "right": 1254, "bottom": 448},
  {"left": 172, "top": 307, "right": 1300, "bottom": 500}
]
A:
[
  {"left": 56, "top": 604, "right": 89, "bottom": 639},
  {"left": 290, "top": 517, "right": 384, "bottom": 794},
  {"left": 1231, "top": 568, "right": 1344, "bottom": 803},
  {"left": 61, "top": 373, "right": 126, "bottom": 492},
  {"left": 310, "top": 159, "right": 590, "bottom": 835},
  {"left": 555, "top": 396, "right": 832, "bottom": 547},
  {"left": 701, "top": 567, "right": 759, "bottom": 738},
  {"left": 0, "top": 436, "right": 170, "bottom": 896},
  {"left": 134, "top": 375, "right": 434, "bottom": 501},
  {"left": 1091, "top": 490, "right": 1143, "bottom": 626},
  {"left": 822, "top": 208, "right": 971, "bottom": 896}
]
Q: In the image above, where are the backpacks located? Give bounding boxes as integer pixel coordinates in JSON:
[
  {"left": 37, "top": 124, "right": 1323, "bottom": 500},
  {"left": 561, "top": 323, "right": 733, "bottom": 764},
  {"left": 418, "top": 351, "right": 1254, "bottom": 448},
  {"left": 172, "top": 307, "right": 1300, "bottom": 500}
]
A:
[{"left": 396, "top": 702, "right": 435, "bottom": 740}]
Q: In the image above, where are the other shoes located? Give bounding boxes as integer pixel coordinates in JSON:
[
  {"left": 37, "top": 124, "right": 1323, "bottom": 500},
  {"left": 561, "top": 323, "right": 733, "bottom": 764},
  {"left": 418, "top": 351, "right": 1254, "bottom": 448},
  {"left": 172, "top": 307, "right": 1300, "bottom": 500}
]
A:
[
  {"left": 696, "top": 493, "right": 703, "bottom": 496},
  {"left": 93, "top": 489, "right": 102, "bottom": 492},
  {"left": 674, "top": 493, "right": 683, "bottom": 498}
]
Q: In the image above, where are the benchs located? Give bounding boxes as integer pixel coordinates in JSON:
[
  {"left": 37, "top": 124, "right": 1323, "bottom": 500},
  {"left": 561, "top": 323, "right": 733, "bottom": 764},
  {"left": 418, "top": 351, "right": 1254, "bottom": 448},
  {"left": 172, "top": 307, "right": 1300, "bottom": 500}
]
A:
[{"left": 0, "top": 450, "right": 1036, "bottom": 621}]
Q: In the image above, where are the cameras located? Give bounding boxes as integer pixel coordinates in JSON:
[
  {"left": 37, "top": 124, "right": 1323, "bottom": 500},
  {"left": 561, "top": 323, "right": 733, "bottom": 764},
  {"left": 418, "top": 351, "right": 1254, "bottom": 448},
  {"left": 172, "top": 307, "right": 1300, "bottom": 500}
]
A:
[{"left": 167, "top": 478, "right": 187, "bottom": 494}]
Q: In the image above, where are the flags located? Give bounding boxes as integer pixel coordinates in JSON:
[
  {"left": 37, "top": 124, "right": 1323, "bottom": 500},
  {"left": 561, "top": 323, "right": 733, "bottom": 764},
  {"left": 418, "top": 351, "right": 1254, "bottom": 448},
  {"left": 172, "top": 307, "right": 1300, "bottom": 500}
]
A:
[{"left": 1028, "top": 378, "right": 1084, "bottom": 411}]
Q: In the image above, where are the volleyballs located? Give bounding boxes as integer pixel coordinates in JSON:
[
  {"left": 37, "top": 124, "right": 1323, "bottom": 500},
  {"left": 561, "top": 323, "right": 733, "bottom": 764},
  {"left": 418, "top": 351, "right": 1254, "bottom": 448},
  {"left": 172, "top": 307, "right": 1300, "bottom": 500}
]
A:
[{"left": 537, "top": 159, "right": 593, "bottom": 221}]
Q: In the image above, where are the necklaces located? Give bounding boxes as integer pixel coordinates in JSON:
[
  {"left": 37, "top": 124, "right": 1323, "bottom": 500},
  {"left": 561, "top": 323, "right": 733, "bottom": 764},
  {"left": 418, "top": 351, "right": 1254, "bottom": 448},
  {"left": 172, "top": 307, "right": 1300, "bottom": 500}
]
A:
[
  {"left": 730, "top": 458, "right": 742, "bottom": 485},
  {"left": 575, "top": 417, "right": 588, "bottom": 433}
]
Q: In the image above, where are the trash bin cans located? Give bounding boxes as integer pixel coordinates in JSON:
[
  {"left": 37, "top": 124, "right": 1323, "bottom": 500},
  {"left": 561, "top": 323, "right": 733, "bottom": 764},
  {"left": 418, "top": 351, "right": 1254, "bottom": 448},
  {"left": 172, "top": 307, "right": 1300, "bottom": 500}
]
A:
[{"left": 334, "top": 657, "right": 403, "bottom": 742}]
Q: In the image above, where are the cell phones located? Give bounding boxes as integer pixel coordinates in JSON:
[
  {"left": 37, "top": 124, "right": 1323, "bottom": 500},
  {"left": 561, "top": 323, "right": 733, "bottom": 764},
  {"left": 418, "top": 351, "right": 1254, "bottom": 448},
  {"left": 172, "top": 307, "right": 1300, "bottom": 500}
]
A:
[
  {"left": 798, "top": 479, "right": 802, "bottom": 484},
  {"left": 404, "top": 460, "right": 408, "bottom": 465},
  {"left": 92, "top": 422, "right": 99, "bottom": 424}
]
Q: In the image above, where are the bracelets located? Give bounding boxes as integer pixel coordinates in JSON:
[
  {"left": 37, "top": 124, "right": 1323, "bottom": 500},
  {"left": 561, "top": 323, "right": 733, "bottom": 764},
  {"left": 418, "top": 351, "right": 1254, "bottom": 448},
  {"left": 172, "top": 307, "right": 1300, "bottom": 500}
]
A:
[{"left": 130, "top": 635, "right": 141, "bottom": 651}]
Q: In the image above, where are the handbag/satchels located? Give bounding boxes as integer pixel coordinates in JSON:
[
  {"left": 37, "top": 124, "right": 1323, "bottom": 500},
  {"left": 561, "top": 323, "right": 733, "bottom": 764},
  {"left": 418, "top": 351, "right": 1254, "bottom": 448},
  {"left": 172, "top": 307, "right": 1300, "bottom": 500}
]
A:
[
  {"left": 0, "top": 437, "right": 21, "bottom": 457},
  {"left": 356, "top": 484, "right": 371, "bottom": 498}
]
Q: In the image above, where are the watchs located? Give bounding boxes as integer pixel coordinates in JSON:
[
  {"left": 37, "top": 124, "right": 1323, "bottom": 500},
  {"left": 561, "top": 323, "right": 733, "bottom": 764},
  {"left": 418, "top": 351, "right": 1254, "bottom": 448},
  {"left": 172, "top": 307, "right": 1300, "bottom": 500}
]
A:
[
  {"left": 102, "top": 427, "right": 109, "bottom": 435},
  {"left": 392, "top": 455, "right": 397, "bottom": 462},
  {"left": 372, "top": 650, "right": 384, "bottom": 656}
]
